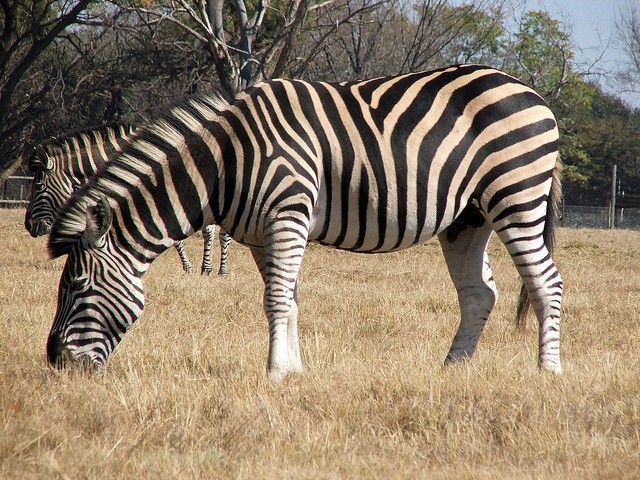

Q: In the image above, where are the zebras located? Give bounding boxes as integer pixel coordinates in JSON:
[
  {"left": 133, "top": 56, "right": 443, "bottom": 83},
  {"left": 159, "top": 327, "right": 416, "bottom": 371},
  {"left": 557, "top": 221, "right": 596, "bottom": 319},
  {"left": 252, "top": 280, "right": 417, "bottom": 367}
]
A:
[
  {"left": 25, "top": 119, "right": 232, "bottom": 278},
  {"left": 46, "top": 63, "right": 566, "bottom": 382}
]
